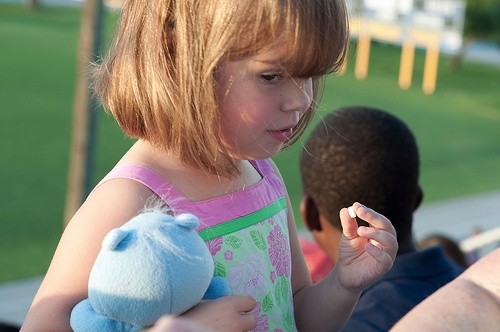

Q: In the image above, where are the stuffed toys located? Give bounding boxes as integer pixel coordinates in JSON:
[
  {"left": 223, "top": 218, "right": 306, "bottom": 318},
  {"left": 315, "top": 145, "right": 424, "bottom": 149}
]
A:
[{"left": 70, "top": 212, "right": 232, "bottom": 332}]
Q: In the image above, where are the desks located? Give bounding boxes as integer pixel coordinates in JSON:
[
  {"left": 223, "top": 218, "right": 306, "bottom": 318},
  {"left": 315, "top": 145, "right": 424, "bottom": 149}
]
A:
[{"left": 337, "top": 17, "right": 443, "bottom": 93}]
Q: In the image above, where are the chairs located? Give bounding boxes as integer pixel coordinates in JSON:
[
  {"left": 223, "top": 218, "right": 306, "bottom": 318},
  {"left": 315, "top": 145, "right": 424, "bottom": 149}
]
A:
[{"left": 424, "top": 0, "right": 467, "bottom": 70}]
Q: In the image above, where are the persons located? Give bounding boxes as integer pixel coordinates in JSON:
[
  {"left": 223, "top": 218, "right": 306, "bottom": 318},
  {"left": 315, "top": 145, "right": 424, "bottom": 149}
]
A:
[
  {"left": 20, "top": 0, "right": 399, "bottom": 332},
  {"left": 299, "top": 105, "right": 465, "bottom": 332}
]
